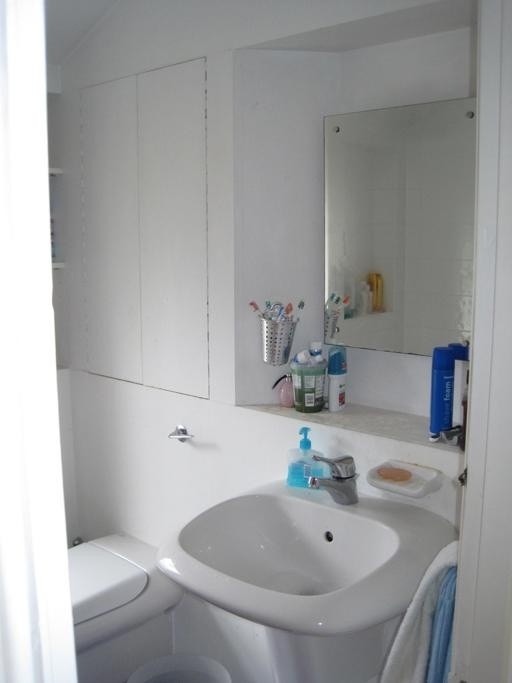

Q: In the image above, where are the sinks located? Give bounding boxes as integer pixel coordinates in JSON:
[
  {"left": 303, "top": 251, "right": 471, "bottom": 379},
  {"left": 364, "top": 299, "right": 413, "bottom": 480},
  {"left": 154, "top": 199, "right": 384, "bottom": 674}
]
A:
[{"left": 155, "top": 477, "right": 460, "bottom": 637}]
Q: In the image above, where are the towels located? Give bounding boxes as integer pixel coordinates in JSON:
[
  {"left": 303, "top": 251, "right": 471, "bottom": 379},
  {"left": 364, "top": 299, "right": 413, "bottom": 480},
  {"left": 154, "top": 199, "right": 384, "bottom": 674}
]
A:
[
  {"left": 425, "top": 565, "right": 457, "bottom": 683},
  {"left": 378, "top": 539, "right": 457, "bottom": 683}
]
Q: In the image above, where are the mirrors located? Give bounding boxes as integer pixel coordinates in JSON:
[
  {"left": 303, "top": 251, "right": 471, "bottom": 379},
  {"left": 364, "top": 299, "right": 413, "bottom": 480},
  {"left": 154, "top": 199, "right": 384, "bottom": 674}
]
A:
[{"left": 322, "top": 96, "right": 479, "bottom": 360}]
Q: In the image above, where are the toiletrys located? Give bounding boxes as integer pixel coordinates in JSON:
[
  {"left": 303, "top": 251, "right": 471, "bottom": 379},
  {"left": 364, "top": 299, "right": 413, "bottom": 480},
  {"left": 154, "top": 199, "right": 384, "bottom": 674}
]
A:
[
  {"left": 359, "top": 272, "right": 383, "bottom": 315},
  {"left": 430, "top": 347, "right": 455, "bottom": 434}
]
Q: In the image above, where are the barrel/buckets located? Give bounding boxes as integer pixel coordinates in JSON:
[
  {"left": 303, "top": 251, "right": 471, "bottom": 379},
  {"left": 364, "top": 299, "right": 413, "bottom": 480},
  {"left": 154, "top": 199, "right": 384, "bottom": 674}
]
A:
[{"left": 126, "top": 655, "right": 233, "bottom": 683}]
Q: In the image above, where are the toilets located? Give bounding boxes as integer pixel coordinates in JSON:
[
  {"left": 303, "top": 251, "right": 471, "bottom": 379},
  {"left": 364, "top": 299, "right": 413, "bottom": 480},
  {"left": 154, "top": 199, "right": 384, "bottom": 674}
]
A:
[{"left": 68, "top": 532, "right": 184, "bottom": 683}]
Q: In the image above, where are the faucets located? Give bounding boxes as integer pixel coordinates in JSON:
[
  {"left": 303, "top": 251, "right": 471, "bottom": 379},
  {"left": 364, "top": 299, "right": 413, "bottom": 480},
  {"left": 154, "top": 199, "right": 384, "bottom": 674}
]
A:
[{"left": 307, "top": 455, "right": 359, "bottom": 506}]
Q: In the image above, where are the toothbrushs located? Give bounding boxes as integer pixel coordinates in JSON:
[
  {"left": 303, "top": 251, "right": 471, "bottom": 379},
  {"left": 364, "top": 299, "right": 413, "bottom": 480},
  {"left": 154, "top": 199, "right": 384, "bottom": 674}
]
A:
[
  {"left": 325, "top": 291, "right": 350, "bottom": 316},
  {"left": 249, "top": 297, "right": 305, "bottom": 322}
]
